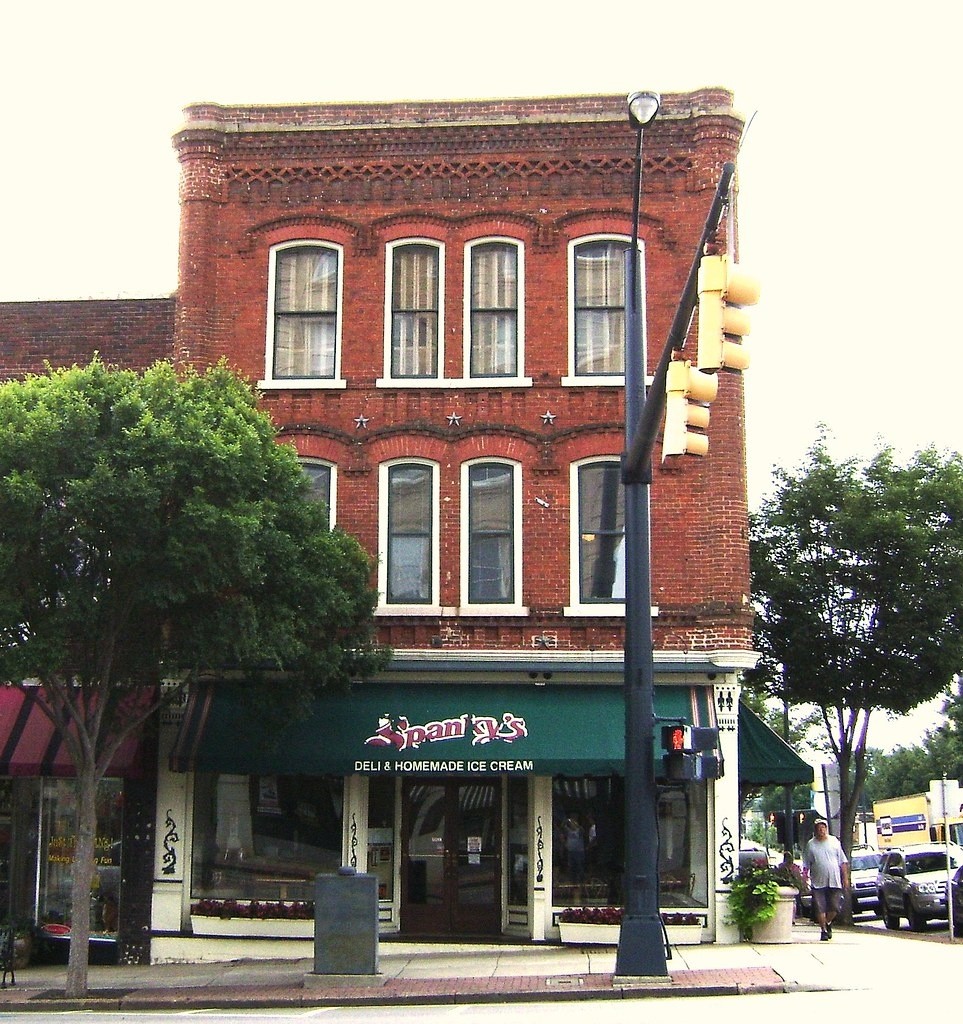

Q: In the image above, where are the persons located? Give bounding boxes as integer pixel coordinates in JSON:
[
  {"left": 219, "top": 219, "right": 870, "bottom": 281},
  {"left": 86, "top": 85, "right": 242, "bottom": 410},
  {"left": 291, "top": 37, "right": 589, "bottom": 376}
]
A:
[{"left": 801, "top": 815, "right": 850, "bottom": 939}]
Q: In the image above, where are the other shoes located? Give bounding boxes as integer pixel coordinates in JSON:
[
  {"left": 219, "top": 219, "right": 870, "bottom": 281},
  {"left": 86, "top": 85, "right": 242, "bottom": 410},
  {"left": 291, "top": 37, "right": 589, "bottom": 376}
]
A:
[
  {"left": 826, "top": 922, "right": 833, "bottom": 939},
  {"left": 821, "top": 931, "right": 828, "bottom": 940}
]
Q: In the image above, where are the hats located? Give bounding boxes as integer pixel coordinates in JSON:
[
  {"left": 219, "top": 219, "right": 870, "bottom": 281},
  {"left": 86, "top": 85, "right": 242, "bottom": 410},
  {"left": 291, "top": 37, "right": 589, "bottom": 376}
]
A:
[{"left": 813, "top": 819, "right": 827, "bottom": 827}]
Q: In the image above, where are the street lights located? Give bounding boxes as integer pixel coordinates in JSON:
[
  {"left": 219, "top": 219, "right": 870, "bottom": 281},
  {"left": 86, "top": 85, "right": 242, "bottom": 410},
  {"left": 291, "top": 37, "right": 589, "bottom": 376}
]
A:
[{"left": 602, "top": 89, "right": 676, "bottom": 987}]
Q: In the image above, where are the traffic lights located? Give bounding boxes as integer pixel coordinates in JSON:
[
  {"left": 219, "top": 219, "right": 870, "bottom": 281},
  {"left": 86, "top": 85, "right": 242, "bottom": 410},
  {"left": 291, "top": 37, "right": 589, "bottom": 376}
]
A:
[
  {"left": 765, "top": 811, "right": 775, "bottom": 823},
  {"left": 689, "top": 723, "right": 726, "bottom": 783},
  {"left": 658, "top": 721, "right": 697, "bottom": 785},
  {"left": 660, "top": 342, "right": 716, "bottom": 458},
  {"left": 794, "top": 812, "right": 805, "bottom": 825},
  {"left": 694, "top": 249, "right": 762, "bottom": 376}
]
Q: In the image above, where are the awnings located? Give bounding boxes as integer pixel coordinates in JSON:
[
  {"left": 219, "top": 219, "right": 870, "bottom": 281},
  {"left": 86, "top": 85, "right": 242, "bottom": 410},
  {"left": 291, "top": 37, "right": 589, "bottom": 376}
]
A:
[
  {"left": 179, "top": 676, "right": 722, "bottom": 783},
  {"left": 0, "top": 681, "right": 153, "bottom": 785},
  {"left": 736, "top": 697, "right": 826, "bottom": 788}
]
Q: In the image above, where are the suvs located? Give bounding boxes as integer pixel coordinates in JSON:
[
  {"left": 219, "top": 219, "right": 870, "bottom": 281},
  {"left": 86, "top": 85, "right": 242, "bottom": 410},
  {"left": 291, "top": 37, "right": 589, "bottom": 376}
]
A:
[
  {"left": 844, "top": 843, "right": 884, "bottom": 921},
  {"left": 876, "top": 842, "right": 963, "bottom": 931}
]
